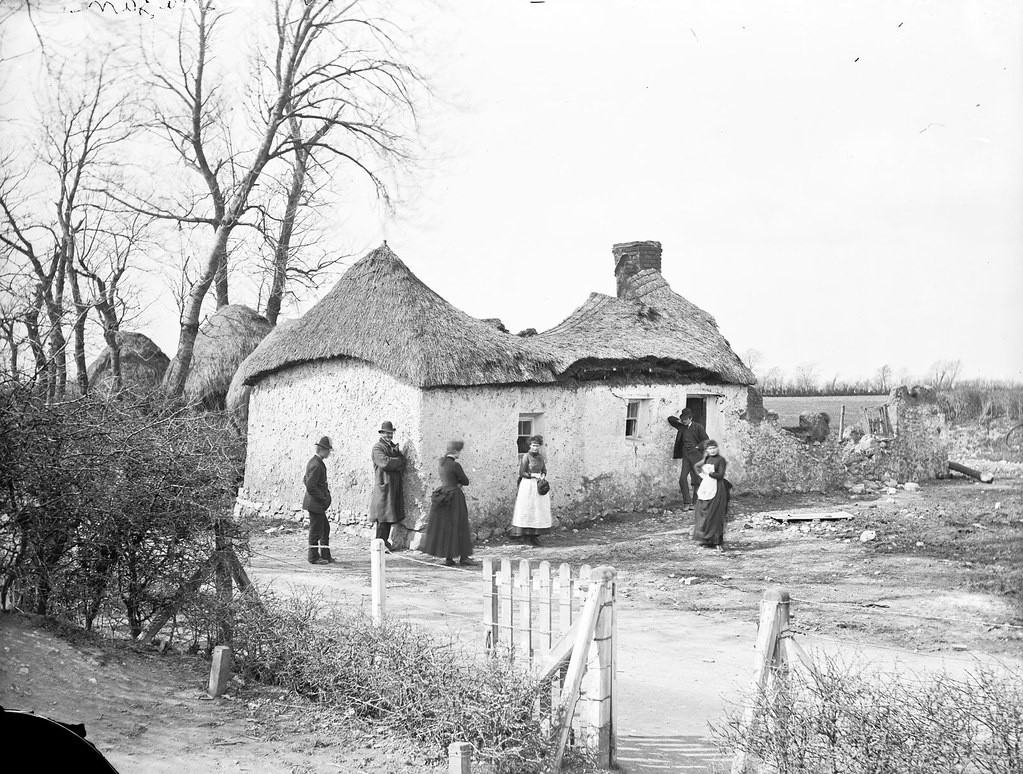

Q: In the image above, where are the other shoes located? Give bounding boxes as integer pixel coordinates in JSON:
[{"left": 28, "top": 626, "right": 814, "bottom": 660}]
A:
[
  {"left": 531, "top": 537, "right": 545, "bottom": 547},
  {"left": 446, "top": 559, "right": 456, "bottom": 566},
  {"left": 384, "top": 540, "right": 395, "bottom": 551},
  {"left": 461, "top": 556, "right": 477, "bottom": 565},
  {"left": 310, "top": 556, "right": 328, "bottom": 564},
  {"left": 683, "top": 502, "right": 696, "bottom": 511},
  {"left": 322, "top": 556, "right": 335, "bottom": 563},
  {"left": 716, "top": 543, "right": 722, "bottom": 552}
]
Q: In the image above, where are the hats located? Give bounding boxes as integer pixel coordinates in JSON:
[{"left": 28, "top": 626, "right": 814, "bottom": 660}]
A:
[
  {"left": 378, "top": 421, "right": 396, "bottom": 433},
  {"left": 680, "top": 408, "right": 692, "bottom": 420},
  {"left": 537, "top": 478, "right": 549, "bottom": 495},
  {"left": 314, "top": 436, "right": 332, "bottom": 449}
]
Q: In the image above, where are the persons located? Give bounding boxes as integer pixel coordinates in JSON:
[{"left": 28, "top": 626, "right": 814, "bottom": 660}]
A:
[
  {"left": 302, "top": 436, "right": 335, "bottom": 564},
  {"left": 370, "top": 421, "right": 406, "bottom": 548},
  {"left": 668, "top": 408, "right": 709, "bottom": 511},
  {"left": 419, "top": 440, "right": 478, "bottom": 565},
  {"left": 513, "top": 435, "right": 551, "bottom": 545},
  {"left": 692, "top": 440, "right": 730, "bottom": 547}
]
